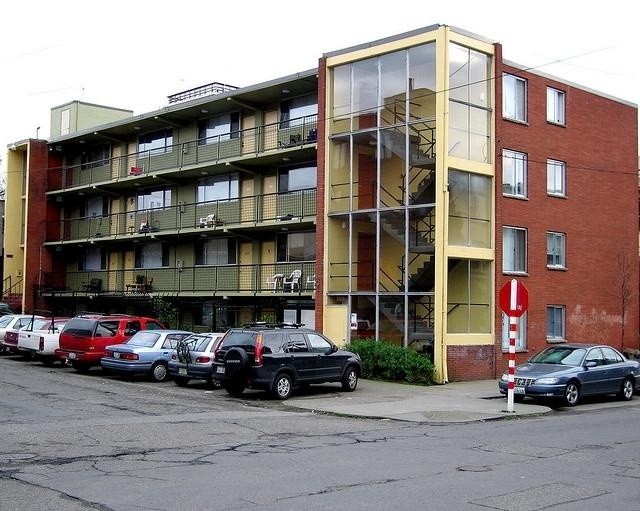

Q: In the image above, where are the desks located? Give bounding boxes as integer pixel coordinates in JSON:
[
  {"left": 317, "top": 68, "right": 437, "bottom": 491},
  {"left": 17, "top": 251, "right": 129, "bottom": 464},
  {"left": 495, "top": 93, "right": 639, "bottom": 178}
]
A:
[
  {"left": 207, "top": 220, "right": 227, "bottom": 229},
  {"left": 126, "top": 284, "right": 145, "bottom": 294}
]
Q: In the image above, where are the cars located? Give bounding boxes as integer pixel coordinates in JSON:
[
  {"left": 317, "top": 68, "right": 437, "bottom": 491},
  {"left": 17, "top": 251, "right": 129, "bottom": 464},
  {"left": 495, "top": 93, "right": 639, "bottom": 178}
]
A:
[
  {"left": 0, "top": 301, "right": 225, "bottom": 392},
  {"left": 498, "top": 343, "right": 640, "bottom": 411}
]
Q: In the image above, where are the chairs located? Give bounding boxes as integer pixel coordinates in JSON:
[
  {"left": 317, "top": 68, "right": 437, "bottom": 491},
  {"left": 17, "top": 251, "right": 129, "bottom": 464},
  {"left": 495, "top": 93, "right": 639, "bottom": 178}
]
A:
[
  {"left": 143, "top": 278, "right": 153, "bottom": 293},
  {"left": 266, "top": 269, "right": 316, "bottom": 293},
  {"left": 80, "top": 279, "right": 101, "bottom": 293},
  {"left": 199, "top": 214, "right": 214, "bottom": 228},
  {"left": 278, "top": 129, "right": 317, "bottom": 149}
]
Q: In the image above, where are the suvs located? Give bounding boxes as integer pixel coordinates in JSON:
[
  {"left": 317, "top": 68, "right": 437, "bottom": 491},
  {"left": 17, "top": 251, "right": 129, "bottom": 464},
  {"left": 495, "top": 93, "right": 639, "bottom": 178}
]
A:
[{"left": 214, "top": 322, "right": 365, "bottom": 401}]
